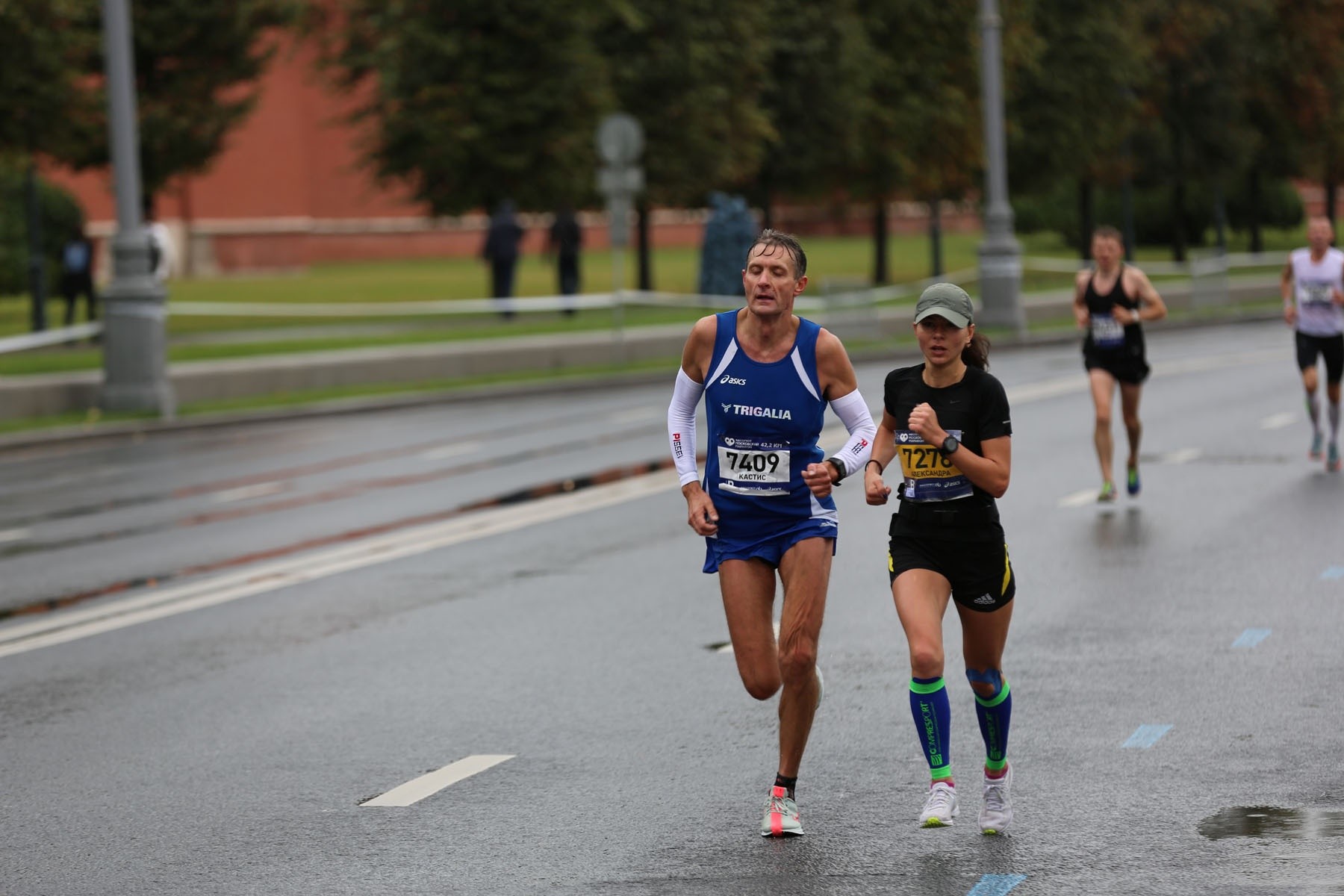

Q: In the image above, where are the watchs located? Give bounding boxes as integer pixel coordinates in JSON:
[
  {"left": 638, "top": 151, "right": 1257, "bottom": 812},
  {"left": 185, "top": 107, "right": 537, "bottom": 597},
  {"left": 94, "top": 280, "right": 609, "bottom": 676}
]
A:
[
  {"left": 938, "top": 434, "right": 959, "bottom": 458},
  {"left": 827, "top": 456, "right": 845, "bottom": 487}
]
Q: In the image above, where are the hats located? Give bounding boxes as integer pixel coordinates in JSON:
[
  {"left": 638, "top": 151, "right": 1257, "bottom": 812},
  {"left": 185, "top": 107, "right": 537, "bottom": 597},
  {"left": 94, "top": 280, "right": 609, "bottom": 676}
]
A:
[{"left": 913, "top": 282, "right": 974, "bottom": 329}]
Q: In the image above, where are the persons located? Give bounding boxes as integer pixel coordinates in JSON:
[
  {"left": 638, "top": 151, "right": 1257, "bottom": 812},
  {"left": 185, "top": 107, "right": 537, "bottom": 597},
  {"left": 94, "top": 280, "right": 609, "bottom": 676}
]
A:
[
  {"left": 1283, "top": 215, "right": 1344, "bottom": 473},
  {"left": 864, "top": 283, "right": 1015, "bottom": 838},
  {"left": 1074, "top": 224, "right": 1166, "bottom": 503},
  {"left": 56, "top": 226, "right": 96, "bottom": 328},
  {"left": 484, "top": 192, "right": 525, "bottom": 321},
  {"left": 667, "top": 232, "right": 876, "bottom": 838},
  {"left": 549, "top": 203, "right": 582, "bottom": 317}
]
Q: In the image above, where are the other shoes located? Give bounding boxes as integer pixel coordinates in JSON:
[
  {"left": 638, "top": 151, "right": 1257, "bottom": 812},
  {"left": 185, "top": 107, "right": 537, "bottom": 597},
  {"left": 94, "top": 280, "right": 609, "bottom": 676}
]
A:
[
  {"left": 1310, "top": 432, "right": 1324, "bottom": 460},
  {"left": 1326, "top": 442, "right": 1341, "bottom": 473}
]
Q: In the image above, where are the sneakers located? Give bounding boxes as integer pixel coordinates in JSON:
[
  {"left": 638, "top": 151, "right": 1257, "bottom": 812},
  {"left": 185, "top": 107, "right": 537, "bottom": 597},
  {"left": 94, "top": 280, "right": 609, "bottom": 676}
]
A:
[
  {"left": 1127, "top": 461, "right": 1140, "bottom": 496},
  {"left": 772, "top": 619, "right": 823, "bottom": 711},
  {"left": 760, "top": 785, "right": 805, "bottom": 837},
  {"left": 918, "top": 780, "right": 960, "bottom": 829},
  {"left": 1097, "top": 480, "right": 1118, "bottom": 502},
  {"left": 977, "top": 761, "right": 1014, "bottom": 835}
]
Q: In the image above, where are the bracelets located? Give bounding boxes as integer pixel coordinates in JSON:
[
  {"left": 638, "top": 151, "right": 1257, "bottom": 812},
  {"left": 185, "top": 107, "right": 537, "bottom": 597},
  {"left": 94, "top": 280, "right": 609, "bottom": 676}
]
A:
[
  {"left": 865, "top": 460, "right": 883, "bottom": 476},
  {"left": 1132, "top": 310, "right": 1140, "bottom": 325},
  {"left": 1285, "top": 299, "right": 1292, "bottom": 308}
]
